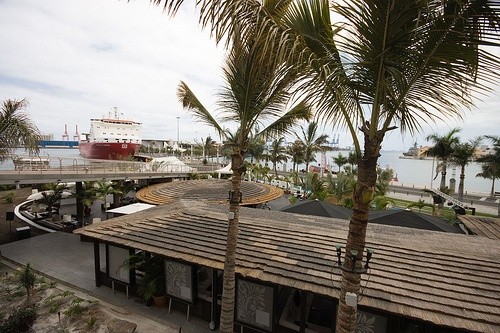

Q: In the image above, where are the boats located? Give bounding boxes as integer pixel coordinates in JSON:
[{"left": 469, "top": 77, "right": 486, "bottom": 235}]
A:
[
  {"left": 79, "top": 106, "right": 142, "bottom": 160},
  {"left": 13, "top": 155, "right": 52, "bottom": 171}
]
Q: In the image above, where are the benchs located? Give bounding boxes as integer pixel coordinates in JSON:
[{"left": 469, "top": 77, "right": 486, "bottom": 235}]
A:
[
  {"left": 279, "top": 288, "right": 332, "bottom": 333},
  {"left": 494, "top": 192, "right": 500, "bottom": 199},
  {"left": 198, "top": 266, "right": 222, "bottom": 305}
]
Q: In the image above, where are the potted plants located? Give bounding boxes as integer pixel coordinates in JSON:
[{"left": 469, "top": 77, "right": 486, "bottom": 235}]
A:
[
  {"left": 72, "top": 181, "right": 103, "bottom": 216},
  {"left": 116, "top": 252, "right": 167, "bottom": 308}
]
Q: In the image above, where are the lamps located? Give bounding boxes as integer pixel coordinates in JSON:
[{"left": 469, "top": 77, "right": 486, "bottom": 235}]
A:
[{"left": 335, "top": 244, "right": 373, "bottom": 274}]
